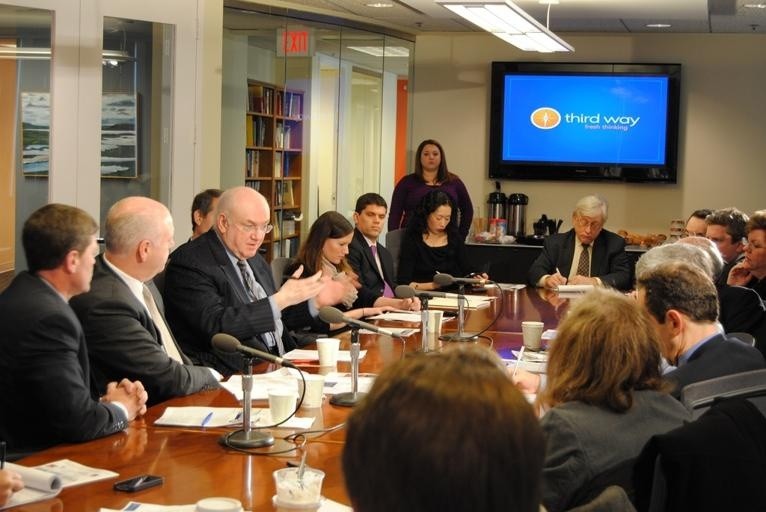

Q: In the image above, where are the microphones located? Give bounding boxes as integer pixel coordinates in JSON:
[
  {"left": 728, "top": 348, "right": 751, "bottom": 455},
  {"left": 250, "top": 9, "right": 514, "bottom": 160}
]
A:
[
  {"left": 318, "top": 306, "right": 402, "bottom": 339},
  {"left": 212, "top": 333, "right": 295, "bottom": 369},
  {"left": 434, "top": 273, "right": 492, "bottom": 286},
  {"left": 394, "top": 285, "right": 467, "bottom": 299}
]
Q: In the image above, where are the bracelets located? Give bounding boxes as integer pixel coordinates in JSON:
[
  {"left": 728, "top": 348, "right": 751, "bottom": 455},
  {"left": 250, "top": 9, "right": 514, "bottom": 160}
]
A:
[{"left": 414, "top": 282, "right": 418, "bottom": 290}]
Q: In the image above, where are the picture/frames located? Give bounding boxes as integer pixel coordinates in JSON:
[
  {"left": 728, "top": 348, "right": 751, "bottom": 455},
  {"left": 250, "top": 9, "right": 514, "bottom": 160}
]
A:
[{"left": 19, "top": 36, "right": 137, "bottom": 181}]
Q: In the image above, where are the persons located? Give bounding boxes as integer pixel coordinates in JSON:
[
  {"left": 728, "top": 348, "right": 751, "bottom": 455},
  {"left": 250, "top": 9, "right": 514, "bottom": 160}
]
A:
[
  {"left": 386, "top": 138, "right": 474, "bottom": 242},
  {"left": 281, "top": 209, "right": 396, "bottom": 337},
  {"left": 637, "top": 260, "right": 765, "bottom": 401},
  {"left": 527, "top": 194, "right": 631, "bottom": 289},
  {"left": 70, "top": 195, "right": 226, "bottom": 405},
  {"left": 1, "top": 203, "right": 149, "bottom": 458},
  {"left": 345, "top": 193, "right": 398, "bottom": 299},
  {"left": 342, "top": 349, "right": 545, "bottom": 512},
  {"left": 1, "top": 469, "right": 22, "bottom": 510},
  {"left": 162, "top": 185, "right": 356, "bottom": 377},
  {"left": 532, "top": 289, "right": 695, "bottom": 512},
  {"left": 400, "top": 190, "right": 488, "bottom": 290},
  {"left": 620, "top": 208, "right": 766, "bottom": 358}
]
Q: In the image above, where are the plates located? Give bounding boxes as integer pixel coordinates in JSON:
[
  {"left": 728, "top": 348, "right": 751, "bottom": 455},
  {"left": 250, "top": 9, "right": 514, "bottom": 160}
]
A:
[{"left": 270, "top": 495, "right": 325, "bottom": 511}]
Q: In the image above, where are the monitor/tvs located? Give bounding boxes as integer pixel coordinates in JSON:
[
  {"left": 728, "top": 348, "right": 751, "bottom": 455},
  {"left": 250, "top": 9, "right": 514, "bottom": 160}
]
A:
[{"left": 490, "top": 60, "right": 682, "bottom": 184}]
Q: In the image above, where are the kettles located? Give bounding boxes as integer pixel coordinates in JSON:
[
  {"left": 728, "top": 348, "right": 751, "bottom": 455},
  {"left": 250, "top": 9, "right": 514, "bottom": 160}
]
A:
[
  {"left": 509, "top": 192, "right": 528, "bottom": 238},
  {"left": 487, "top": 192, "right": 508, "bottom": 219}
]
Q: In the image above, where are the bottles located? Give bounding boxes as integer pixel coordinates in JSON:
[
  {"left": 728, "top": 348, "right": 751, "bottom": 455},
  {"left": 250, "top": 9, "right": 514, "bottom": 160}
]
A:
[
  {"left": 490, "top": 218, "right": 496, "bottom": 235},
  {"left": 495, "top": 219, "right": 506, "bottom": 238},
  {"left": 474, "top": 217, "right": 483, "bottom": 236}
]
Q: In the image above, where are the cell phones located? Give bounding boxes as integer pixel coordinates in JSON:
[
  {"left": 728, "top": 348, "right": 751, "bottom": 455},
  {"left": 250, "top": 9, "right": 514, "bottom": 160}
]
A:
[{"left": 114, "top": 474, "right": 163, "bottom": 492}]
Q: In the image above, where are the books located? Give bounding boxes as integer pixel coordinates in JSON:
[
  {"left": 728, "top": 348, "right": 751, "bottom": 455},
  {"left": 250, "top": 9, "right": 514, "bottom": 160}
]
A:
[
  {"left": 245, "top": 79, "right": 301, "bottom": 264},
  {"left": 1, "top": 459, "right": 64, "bottom": 510}
]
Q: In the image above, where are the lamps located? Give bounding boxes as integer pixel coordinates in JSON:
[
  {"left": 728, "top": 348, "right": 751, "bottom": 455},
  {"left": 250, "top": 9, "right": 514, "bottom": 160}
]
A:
[{"left": 435, "top": 0, "right": 575, "bottom": 55}]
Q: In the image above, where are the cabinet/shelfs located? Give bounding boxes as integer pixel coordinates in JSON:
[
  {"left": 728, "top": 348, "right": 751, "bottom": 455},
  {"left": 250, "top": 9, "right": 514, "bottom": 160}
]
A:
[{"left": 246, "top": 77, "right": 306, "bottom": 265}]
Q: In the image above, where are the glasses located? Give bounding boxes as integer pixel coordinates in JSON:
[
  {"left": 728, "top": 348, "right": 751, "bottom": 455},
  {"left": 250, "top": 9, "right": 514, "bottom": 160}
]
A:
[
  {"left": 741, "top": 236, "right": 748, "bottom": 245},
  {"left": 579, "top": 219, "right": 600, "bottom": 231},
  {"left": 239, "top": 224, "right": 274, "bottom": 234}
]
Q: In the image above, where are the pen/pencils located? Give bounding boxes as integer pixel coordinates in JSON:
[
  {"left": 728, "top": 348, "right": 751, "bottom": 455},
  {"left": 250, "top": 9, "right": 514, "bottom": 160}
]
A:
[
  {"left": 410, "top": 295, "right": 415, "bottom": 303},
  {"left": 201, "top": 412, "right": 213, "bottom": 426},
  {"left": 555, "top": 267, "right": 564, "bottom": 277},
  {"left": 280, "top": 274, "right": 325, "bottom": 283},
  {"left": 512, "top": 345, "right": 526, "bottom": 378},
  {"left": 0, "top": 441, "right": 7, "bottom": 469},
  {"left": 435, "top": 270, "right": 441, "bottom": 276}
]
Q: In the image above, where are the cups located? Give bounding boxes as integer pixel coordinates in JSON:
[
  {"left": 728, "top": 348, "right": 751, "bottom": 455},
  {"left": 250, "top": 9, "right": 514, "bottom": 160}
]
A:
[
  {"left": 271, "top": 465, "right": 325, "bottom": 501},
  {"left": 267, "top": 389, "right": 298, "bottom": 425},
  {"left": 314, "top": 337, "right": 342, "bottom": 367},
  {"left": 522, "top": 321, "right": 544, "bottom": 351},
  {"left": 424, "top": 310, "right": 445, "bottom": 335},
  {"left": 297, "top": 375, "right": 326, "bottom": 408}
]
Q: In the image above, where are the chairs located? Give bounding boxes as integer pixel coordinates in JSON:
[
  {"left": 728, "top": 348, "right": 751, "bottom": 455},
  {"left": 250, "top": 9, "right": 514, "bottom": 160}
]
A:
[
  {"left": 270, "top": 257, "right": 288, "bottom": 292},
  {"left": 630, "top": 332, "right": 766, "bottom": 512},
  {"left": 385, "top": 227, "right": 403, "bottom": 278}
]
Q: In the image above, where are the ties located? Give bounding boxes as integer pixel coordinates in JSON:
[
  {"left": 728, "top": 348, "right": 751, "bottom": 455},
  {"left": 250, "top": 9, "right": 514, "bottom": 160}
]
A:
[
  {"left": 371, "top": 246, "right": 394, "bottom": 298},
  {"left": 237, "top": 261, "right": 285, "bottom": 357},
  {"left": 143, "top": 285, "right": 184, "bottom": 365},
  {"left": 576, "top": 243, "right": 589, "bottom": 277}
]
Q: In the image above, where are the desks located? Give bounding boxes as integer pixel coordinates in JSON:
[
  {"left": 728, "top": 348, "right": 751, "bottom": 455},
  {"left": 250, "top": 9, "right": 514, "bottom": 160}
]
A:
[
  {"left": 0, "top": 283, "right": 584, "bottom": 512},
  {"left": 463, "top": 237, "right": 651, "bottom": 291}
]
